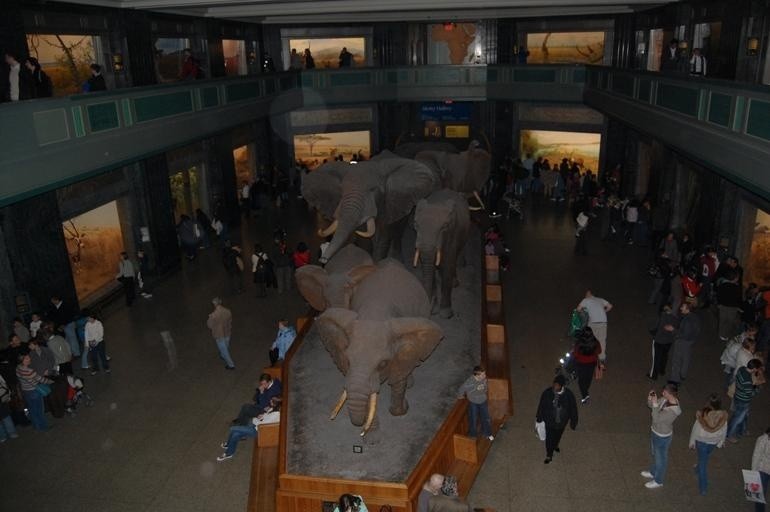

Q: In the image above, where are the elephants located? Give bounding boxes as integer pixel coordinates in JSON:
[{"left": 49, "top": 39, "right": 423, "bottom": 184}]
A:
[
  {"left": 293, "top": 139, "right": 492, "bottom": 321},
  {"left": 317, "top": 257, "right": 446, "bottom": 437}
]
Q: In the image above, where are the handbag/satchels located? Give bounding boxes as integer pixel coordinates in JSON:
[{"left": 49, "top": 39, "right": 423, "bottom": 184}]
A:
[
  {"left": 592, "top": 363, "right": 603, "bottom": 382},
  {"left": 740, "top": 468, "right": 767, "bottom": 505},
  {"left": 534, "top": 419, "right": 547, "bottom": 441},
  {"left": 34, "top": 382, "right": 53, "bottom": 397}
]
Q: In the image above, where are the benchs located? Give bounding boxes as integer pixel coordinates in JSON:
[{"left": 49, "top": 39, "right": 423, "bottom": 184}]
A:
[
  {"left": 243, "top": 311, "right": 317, "bottom": 511},
  {"left": 408, "top": 253, "right": 516, "bottom": 511}
]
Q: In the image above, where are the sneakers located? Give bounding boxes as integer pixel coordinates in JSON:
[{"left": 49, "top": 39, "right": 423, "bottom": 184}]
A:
[
  {"left": 640, "top": 470, "right": 655, "bottom": 479},
  {"left": 221, "top": 441, "right": 230, "bottom": 449},
  {"left": 667, "top": 374, "right": 685, "bottom": 385},
  {"left": 644, "top": 480, "right": 664, "bottom": 489},
  {"left": 739, "top": 431, "right": 754, "bottom": 437},
  {"left": 544, "top": 454, "right": 553, "bottom": 464},
  {"left": 554, "top": 446, "right": 560, "bottom": 453},
  {"left": 0, "top": 433, "right": 22, "bottom": 442},
  {"left": 90, "top": 369, "right": 97, "bottom": 375},
  {"left": 139, "top": 291, "right": 153, "bottom": 298},
  {"left": 216, "top": 452, "right": 233, "bottom": 461},
  {"left": 482, "top": 435, "right": 495, "bottom": 441},
  {"left": 580, "top": 394, "right": 590, "bottom": 403},
  {"left": 725, "top": 436, "right": 738, "bottom": 443},
  {"left": 104, "top": 368, "right": 112, "bottom": 376},
  {"left": 224, "top": 364, "right": 235, "bottom": 369},
  {"left": 647, "top": 369, "right": 665, "bottom": 380}
]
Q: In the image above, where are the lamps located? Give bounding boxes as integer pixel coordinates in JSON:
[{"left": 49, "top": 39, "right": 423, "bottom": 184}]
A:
[
  {"left": 678, "top": 38, "right": 689, "bottom": 57},
  {"left": 745, "top": 36, "right": 759, "bottom": 58},
  {"left": 110, "top": 51, "right": 125, "bottom": 75}
]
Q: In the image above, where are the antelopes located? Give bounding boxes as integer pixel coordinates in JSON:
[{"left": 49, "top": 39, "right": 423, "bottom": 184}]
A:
[
  {"left": 63, "top": 219, "right": 85, "bottom": 273},
  {"left": 566, "top": 149, "right": 586, "bottom": 171}
]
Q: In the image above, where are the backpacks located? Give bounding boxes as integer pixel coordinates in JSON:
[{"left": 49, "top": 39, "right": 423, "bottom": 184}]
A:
[{"left": 257, "top": 258, "right": 273, "bottom": 277}]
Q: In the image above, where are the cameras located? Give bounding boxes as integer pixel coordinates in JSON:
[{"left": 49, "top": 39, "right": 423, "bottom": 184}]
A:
[{"left": 650, "top": 391, "right": 655, "bottom": 396}]
